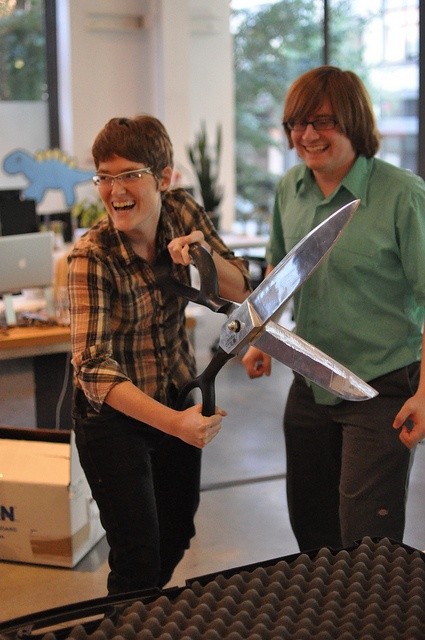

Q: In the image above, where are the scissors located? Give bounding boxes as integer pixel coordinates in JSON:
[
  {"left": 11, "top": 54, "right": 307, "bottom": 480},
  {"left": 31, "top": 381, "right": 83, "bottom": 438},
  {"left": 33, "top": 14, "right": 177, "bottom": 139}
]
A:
[{"left": 163, "top": 199, "right": 380, "bottom": 418}]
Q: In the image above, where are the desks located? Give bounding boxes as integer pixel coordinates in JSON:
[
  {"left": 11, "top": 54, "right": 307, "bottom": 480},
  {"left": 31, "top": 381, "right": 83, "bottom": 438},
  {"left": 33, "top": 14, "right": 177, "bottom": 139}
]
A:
[{"left": 0, "top": 308, "right": 198, "bottom": 428}]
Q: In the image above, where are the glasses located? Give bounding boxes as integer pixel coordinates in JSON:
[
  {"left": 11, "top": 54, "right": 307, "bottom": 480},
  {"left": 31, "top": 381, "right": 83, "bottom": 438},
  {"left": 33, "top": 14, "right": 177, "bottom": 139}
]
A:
[
  {"left": 91, "top": 166, "right": 154, "bottom": 188},
  {"left": 282, "top": 115, "right": 338, "bottom": 132}
]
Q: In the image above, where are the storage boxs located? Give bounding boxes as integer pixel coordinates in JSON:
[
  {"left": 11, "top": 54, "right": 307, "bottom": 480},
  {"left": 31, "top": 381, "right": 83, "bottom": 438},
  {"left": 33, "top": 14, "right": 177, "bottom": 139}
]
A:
[{"left": 0, "top": 425, "right": 106, "bottom": 569}]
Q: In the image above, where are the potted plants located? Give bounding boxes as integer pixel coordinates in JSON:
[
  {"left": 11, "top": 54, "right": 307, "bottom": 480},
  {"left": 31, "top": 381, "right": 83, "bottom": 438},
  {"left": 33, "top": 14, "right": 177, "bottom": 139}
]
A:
[{"left": 183, "top": 118, "right": 225, "bottom": 236}]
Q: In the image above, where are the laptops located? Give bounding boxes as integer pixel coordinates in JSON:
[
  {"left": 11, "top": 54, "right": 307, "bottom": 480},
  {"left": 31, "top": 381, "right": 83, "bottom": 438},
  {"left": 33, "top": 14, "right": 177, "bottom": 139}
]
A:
[{"left": 0, "top": 231, "right": 56, "bottom": 296}]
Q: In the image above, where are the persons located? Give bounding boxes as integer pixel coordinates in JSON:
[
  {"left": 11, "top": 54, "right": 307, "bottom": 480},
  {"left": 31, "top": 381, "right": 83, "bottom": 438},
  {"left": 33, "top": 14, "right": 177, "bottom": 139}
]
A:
[
  {"left": 242, "top": 67, "right": 425, "bottom": 553},
  {"left": 68, "top": 114, "right": 252, "bottom": 596}
]
